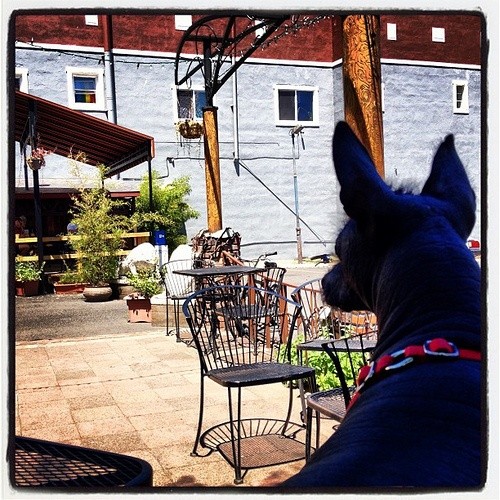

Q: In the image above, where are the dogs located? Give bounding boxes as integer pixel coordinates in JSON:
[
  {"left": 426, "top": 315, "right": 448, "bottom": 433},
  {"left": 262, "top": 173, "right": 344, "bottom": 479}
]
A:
[{"left": 275, "top": 120, "right": 482, "bottom": 494}]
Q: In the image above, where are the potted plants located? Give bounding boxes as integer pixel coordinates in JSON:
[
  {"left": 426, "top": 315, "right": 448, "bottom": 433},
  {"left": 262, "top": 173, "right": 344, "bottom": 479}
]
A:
[
  {"left": 54, "top": 266, "right": 88, "bottom": 296},
  {"left": 68, "top": 145, "right": 128, "bottom": 300},
  {"left": 176, "top": 122, "right": 203, "bottom": 169},
  {"left": 125, "top": 270, "right": 163, "bottom": 323},
  {"left": 14, "top": 255, "right": 44, "bottom": 295},
  {"left": 25, "top": 146, "right": 48, "bottom": 171}
]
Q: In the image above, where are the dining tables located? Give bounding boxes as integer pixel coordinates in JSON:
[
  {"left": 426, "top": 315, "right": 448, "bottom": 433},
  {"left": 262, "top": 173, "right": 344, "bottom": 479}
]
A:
[{"left": 171, "top": 266, "right": 269, "bottom": 361}]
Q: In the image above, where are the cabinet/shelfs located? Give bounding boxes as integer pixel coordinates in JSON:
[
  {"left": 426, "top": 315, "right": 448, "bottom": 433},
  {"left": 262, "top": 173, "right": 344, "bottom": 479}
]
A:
[{"left": 14, "top": 215, "right": 150, "bottom": 292}]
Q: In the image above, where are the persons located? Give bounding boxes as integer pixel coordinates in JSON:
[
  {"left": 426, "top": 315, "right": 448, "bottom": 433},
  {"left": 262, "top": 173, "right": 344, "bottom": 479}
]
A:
[{"left": 14, "top": 216, "right": 36, "bottom": 262}]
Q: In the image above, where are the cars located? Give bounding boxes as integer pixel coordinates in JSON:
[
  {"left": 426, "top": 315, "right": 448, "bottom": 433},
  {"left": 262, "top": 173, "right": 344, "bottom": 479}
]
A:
[{"left": 465, "top": 237, "right": 481, "bottom": 255}]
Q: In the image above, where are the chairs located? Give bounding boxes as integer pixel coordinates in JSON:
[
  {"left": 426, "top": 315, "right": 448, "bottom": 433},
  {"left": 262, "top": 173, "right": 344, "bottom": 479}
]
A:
[
  {"left": 161, "top": 258, "right": 373, "bottom": 484},
  {"left": 12, "top": 434, "right": 154, "bottom": 487}
]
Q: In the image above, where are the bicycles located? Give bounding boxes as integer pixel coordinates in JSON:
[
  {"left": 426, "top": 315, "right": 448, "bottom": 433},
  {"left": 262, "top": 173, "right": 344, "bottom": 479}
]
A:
[
  {"left": 234, "top": 252, "right": 285, "bottom": 321},
  {"left": 310, "top": 253, "right": 376, "bottom": 336}
]
